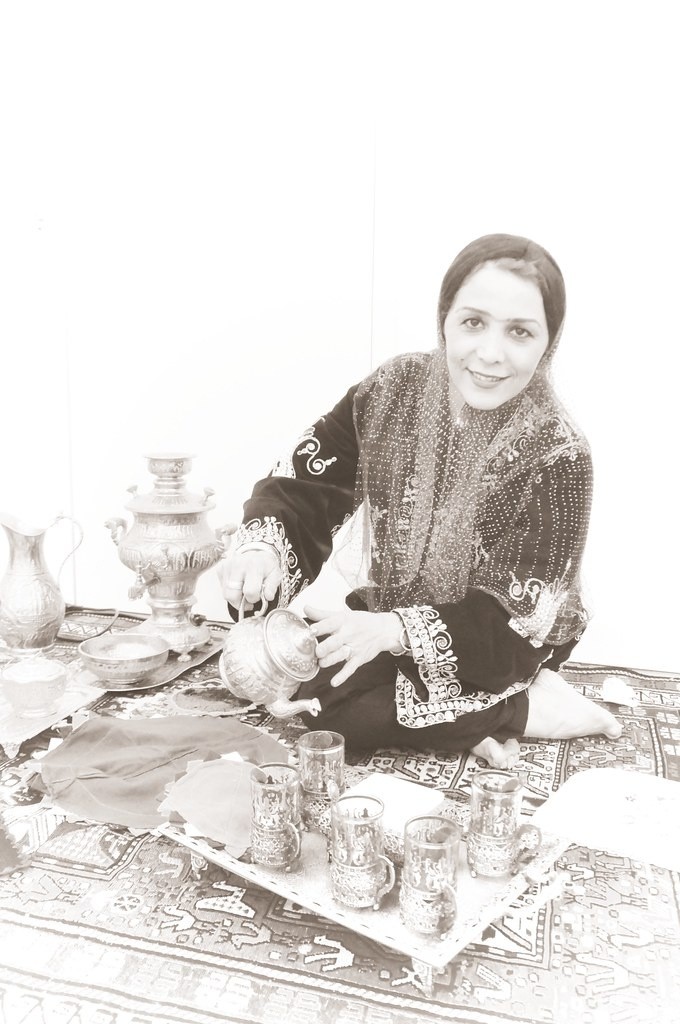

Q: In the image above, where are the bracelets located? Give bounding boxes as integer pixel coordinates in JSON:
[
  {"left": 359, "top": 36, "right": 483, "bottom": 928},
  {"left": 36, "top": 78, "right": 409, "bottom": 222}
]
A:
[
  {"left": 389, "top": 644, "right": 410, "bottom": 656},
  {"left": 238, "top": 541, "right": 281, "bottom": 568}
]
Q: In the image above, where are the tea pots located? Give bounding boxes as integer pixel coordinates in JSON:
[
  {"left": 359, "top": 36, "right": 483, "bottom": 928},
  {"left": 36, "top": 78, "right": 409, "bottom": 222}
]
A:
[{"left": 219, "top": 592, "right": 321, "bottom": 718}]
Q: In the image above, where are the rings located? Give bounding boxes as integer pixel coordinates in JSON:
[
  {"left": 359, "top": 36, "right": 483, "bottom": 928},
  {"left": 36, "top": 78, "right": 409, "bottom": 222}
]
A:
[{"left": 344, "top": 642, "right": 354, "bottom": 661}]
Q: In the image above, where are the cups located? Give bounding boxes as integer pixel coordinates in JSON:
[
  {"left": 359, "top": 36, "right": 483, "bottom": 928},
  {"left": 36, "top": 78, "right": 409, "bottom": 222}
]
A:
[
  {"left": 297, "top": 730, "right": 345, "bottom": 833},
  {"left": 465, "top": 771, "right": 541, "bottom": 877},
  {"left": 330, "top": 795, "right": 396, "bottom": 910},
  {"left": 251, "top": 763, "right": 302, "bottom": 873},
  {"left": 399, "top": 816, "right": 461, "bottom": 941},
  {"left": 4, "top": 660, "right": 67, "bottom": 718}
]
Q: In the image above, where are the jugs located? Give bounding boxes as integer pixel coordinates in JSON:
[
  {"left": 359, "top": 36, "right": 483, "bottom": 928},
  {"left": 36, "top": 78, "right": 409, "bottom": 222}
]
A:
[{"left": 0, "top": 512, "right": 84, "bottom": 655}]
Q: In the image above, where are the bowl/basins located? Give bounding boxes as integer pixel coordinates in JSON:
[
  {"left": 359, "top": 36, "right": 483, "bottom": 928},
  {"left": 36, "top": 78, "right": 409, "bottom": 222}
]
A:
[{"left": 78, "top": 634, "right": 169, "bottom": 685}]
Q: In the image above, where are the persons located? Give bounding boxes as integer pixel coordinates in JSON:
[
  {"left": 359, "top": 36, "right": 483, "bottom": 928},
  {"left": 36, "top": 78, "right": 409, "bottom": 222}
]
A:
[{"left": 214, "top": 232, "right": 624, "bottom": 770}]
